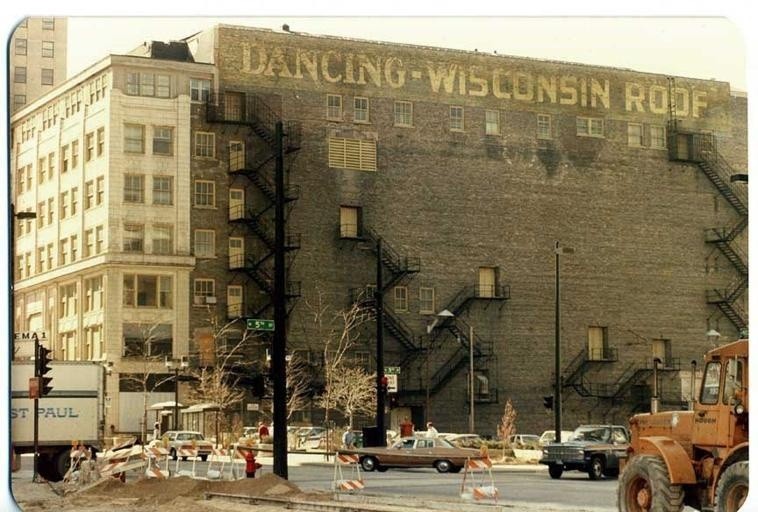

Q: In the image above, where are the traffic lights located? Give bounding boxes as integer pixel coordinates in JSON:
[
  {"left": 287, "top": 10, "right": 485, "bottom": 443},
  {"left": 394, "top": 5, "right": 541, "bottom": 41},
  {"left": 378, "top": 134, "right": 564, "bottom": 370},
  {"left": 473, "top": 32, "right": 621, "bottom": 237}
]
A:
[
  {"left": 39, "top": 345, "right": 52, "bottom": 395},
  {"left": 377, "top": 375, "right": 389, "bottom": 398}
]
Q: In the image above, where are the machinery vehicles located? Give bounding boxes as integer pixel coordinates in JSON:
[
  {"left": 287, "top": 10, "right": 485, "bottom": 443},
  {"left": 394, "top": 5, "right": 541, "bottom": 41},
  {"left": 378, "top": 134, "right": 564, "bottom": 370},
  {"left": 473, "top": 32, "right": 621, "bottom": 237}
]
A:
[{"left": 618, "top": 339, "right": 748, "bottom": 512}]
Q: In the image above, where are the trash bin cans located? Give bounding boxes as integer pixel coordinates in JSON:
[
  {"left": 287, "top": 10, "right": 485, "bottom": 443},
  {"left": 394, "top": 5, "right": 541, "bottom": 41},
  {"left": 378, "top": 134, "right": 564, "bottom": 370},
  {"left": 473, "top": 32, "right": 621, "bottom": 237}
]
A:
[{"left": 362, "top": 426, "right": 387, "bottom": 447}]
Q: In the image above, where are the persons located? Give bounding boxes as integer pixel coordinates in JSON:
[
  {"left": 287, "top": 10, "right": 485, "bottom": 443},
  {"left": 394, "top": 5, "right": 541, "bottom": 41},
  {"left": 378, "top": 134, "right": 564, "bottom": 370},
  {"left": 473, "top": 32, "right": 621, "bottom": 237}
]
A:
[
  {"left": 258, "top": 422, "right": 268, "bottom": 443},
  {"left": 342, "top": 426, "right": 352, "bottom": 450},
  {"left": 153, "top": 422, "right": 159, "bottom": 440},
  {"left": 426, "top": 421, "right": 438, "bottom": 433}
]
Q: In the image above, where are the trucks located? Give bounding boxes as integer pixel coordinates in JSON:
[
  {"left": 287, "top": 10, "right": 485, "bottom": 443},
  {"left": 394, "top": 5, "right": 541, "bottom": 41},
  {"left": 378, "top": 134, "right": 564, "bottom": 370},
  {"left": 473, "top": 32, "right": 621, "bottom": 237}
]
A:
[{"left": 11, "top": 360, "right": 111, "bottom": 482}]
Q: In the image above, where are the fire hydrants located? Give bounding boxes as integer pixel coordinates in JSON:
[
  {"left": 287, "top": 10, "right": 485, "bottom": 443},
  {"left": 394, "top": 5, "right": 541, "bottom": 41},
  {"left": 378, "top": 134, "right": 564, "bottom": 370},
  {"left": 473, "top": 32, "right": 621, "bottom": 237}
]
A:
[{"left": 244, "top": 450, "right": 263, "bottom": 478}]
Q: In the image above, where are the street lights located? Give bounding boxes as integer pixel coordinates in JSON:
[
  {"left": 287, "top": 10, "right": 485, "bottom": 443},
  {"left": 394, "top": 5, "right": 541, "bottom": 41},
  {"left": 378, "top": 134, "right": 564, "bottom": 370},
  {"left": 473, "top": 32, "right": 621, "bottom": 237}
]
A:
[
  {"left": 553, "top": 241, "right": 574, "bottom": 441},
  {"left": 165, "top": 355, "right": 189, "bottom": 430},
  {"left": 11, "top": 204, "right": 36, "bottom": 361}
]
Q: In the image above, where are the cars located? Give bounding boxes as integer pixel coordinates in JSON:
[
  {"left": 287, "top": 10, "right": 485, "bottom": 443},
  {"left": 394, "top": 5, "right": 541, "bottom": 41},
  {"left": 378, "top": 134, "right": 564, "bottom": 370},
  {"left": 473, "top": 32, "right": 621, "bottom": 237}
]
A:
[
  {"left": 162, "top": 431, "right": 212, "bottom": 461},
  {"left": 244, "top": 426, "right": 325, "bottom": 451},
  {"left": 510, "top": 425, "right": 629, "bottom": 480},
  {"left": 358, "top": 430, "right": 481, "bottom": 473}
]
